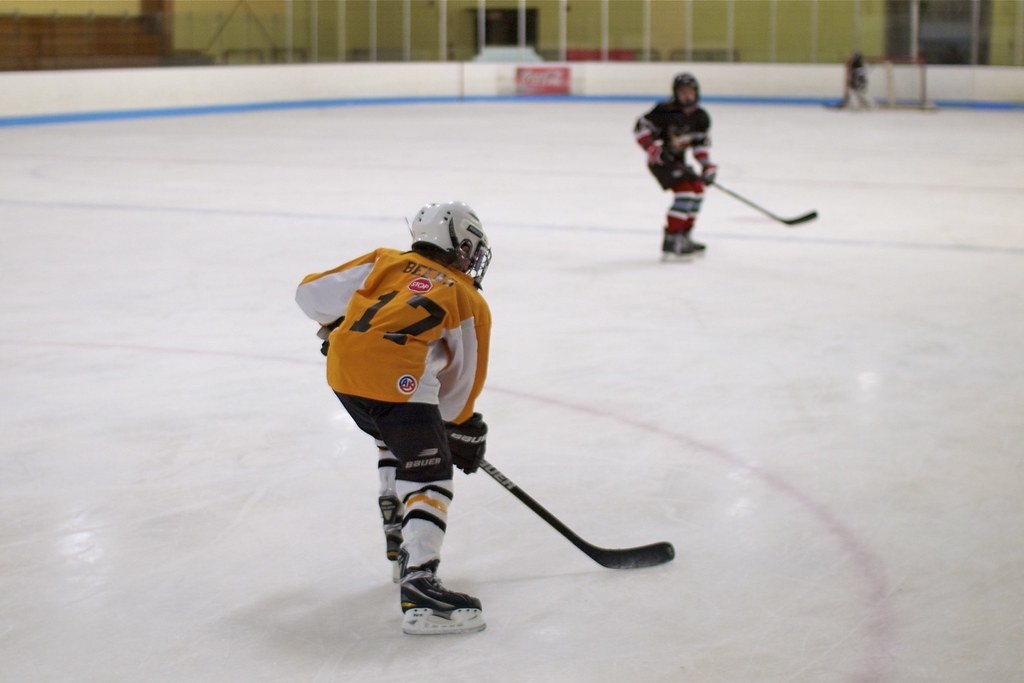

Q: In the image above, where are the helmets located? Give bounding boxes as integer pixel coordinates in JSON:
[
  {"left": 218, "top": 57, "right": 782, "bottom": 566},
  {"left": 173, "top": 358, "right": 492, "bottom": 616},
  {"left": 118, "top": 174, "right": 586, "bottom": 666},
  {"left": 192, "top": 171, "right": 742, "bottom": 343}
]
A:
[
  {"left": 412, "top": 200, "right": 493, "bottom": 288},
  {"left": 673, "top": 74, "right": 700, "bottom": 107}
]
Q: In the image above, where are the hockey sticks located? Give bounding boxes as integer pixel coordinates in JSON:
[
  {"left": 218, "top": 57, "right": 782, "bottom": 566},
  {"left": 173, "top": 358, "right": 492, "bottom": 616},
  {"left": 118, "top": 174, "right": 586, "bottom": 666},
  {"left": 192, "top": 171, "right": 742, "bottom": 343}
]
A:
[
  {"left": 669, "top": 157, "right": 817, "bottom": 225},
  {"left": 317, "top": 322, "right": 675, "bottom": 569}
]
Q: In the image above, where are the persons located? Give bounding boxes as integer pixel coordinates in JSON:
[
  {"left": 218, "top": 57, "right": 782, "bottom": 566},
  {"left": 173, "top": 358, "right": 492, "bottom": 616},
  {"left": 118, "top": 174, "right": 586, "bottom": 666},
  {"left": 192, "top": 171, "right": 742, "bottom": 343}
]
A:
[
  {"left": 296, "top": 202, "right": 498, "bottom": 638},
  {"left": 635, "top": 75, "right": 715, "bottom": 262},
  {"left": 846, "top": 52, "right": 870, "bottom": 111}
]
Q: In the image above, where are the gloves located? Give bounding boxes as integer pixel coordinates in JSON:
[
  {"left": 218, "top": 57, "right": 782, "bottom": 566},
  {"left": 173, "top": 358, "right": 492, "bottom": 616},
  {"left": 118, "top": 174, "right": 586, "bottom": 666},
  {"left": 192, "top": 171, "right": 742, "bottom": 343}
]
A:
[{"left": 446, "top": 412, "right": 488, "bottom": 475}]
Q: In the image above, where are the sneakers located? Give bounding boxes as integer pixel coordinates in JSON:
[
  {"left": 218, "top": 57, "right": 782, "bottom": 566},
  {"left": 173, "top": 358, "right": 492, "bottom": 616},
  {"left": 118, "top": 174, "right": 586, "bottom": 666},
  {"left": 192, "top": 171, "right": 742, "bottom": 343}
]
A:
[
  {"left": 684, "top": 235, "right": 705, "bottom": 256},
  {"left": 662, "top": 232, "right": 696, "bottom": 261},
  {"left": 395, "top": 545, "right": 487, "bottom": 634},
  {"left": 378, "top": 494, "right": 406, "bottom": 559}
]
